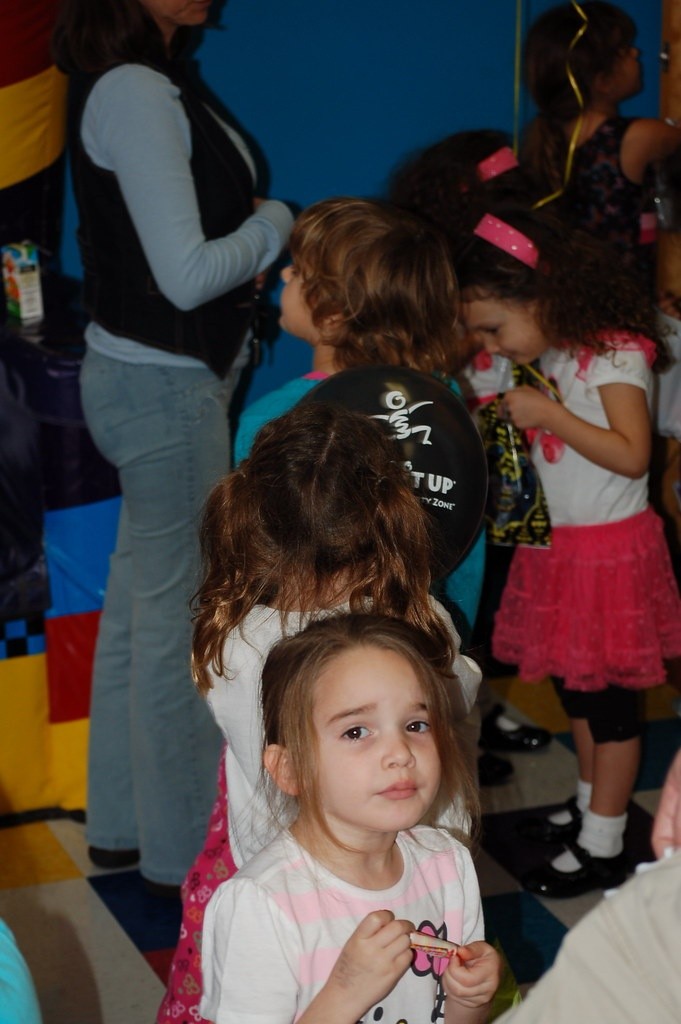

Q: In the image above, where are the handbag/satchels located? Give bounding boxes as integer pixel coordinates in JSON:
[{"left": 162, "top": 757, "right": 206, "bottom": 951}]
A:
[{"left": 472, "top": 391, "right": 553, "bottom": 550}]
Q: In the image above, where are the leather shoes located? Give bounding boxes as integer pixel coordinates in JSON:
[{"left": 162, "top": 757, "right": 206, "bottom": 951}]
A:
[
  {"left": 478, "top": 751, "right": 513, "bottom": 787},
  {"left": 481, "top": 704, "right": 552, "bottom": 751},
  {"left": 516, "top": 796, "right": 583, "bottom": 845},
  {"left": 526, "top": 841, "right": 626, "bottom": 897}
]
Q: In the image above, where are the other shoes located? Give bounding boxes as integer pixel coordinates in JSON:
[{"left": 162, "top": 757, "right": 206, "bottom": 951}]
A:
[
  {"left": 142, "top": 878, "right": 183, "bottom": 899},
  {"left": 87, "top": 845, "right": 141, "bottom": 868}
]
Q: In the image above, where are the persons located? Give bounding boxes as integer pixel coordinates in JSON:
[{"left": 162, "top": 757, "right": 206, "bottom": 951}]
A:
[
  {"left": 197, "top": 614, "right": 501, "bottom": 1024},
  {"left": 232, "top": 199, "right": 486, "bottom": 643},
  {"left": 383, "top": 0, "right": 681, "bottom": 898},
  {"left": 158, "top": 366, "right": 490, "bottom": 1024},
  {"left": 53, "top": 1, "right": 299, "bottom": 889}
]
